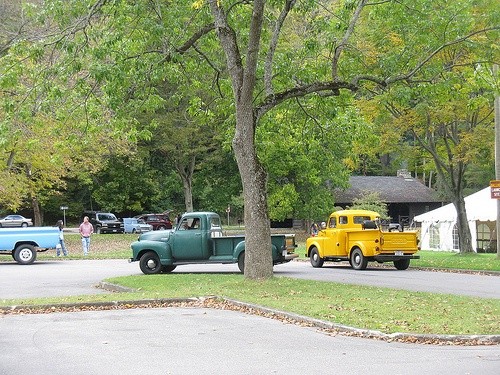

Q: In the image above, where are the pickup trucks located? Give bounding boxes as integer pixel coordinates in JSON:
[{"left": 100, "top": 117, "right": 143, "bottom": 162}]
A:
[
  {"left": 0, "top": 226, "right": 61, "bottom": 265},
  {"left": 128, "top": 211, "right": 300, "bottom": 274},
  {"left": 304, "top": 209, "right": 422, "bottom": 270}
]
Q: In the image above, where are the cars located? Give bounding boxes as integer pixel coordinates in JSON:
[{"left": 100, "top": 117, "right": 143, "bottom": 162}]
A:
[{"left": 0, "top": 215, "right": 33, "bottom": 227}]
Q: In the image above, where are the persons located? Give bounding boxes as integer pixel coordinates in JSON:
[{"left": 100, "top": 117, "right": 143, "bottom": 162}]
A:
[
  {"left": 55, "top": 220, "right": 67, "bottom": 257},
  {"left": 174, "top": 213, "right": 181, "bottom": 227},
  {"left": 311, "top": 220, "right": 329, "bottom": 235},
  {"left": 400, "top": 217, "right": 406, "bottom": 231},
  {"left": 79, "top": 216, "right": 94, "bottom": 255},
  {"left": 186, "top": 219, "right": 200, "bottom": 230}
]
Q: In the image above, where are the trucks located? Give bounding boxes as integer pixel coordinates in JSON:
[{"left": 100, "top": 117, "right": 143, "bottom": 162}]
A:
[{"left": 118, "top": 217, "right": 153, "bottom": 235}]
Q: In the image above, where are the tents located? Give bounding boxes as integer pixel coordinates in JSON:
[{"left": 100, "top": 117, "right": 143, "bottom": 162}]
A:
[{"left": 414, "top": 186, "right": 497, "bottom": 250}]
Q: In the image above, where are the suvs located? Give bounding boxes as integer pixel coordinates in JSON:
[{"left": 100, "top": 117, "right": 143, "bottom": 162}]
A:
[
  {"left": 138, "top": 213, "right": 173, "bottom": 230},
  {"left": 78, "top": 210, "right": 125, "bottom": 235}
]
